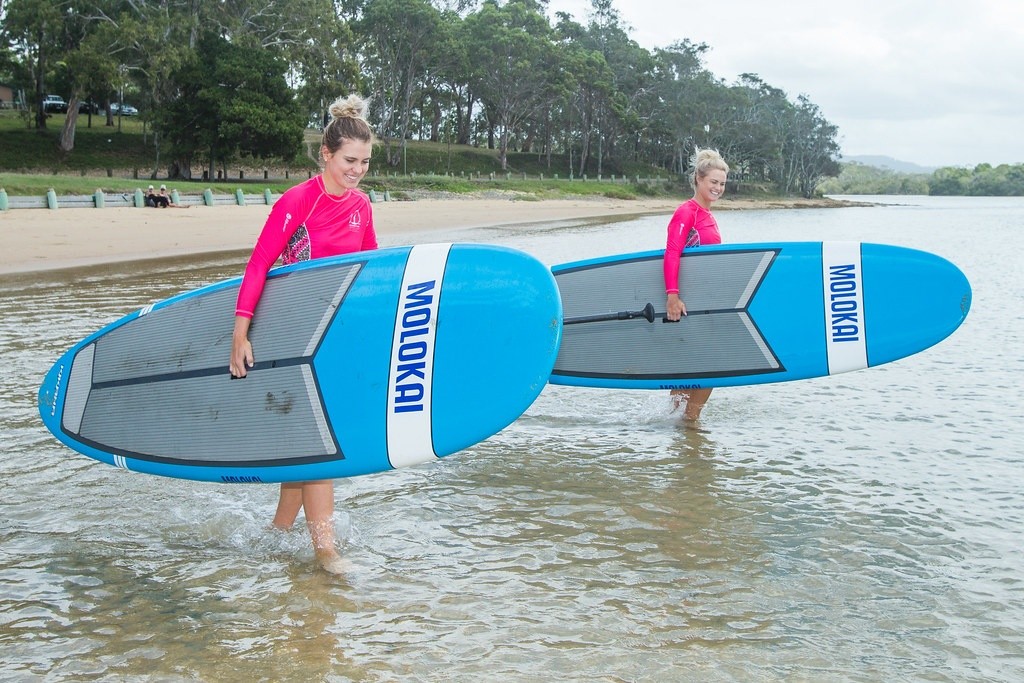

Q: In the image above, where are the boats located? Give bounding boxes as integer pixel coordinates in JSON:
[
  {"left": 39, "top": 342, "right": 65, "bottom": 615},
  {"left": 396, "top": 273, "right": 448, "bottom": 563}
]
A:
[
  {"left": 549, "top": 238, "right": 973, "bottom": 390},
  {"left": 37, "top": 241, "right": 564, "bottom": 486}
]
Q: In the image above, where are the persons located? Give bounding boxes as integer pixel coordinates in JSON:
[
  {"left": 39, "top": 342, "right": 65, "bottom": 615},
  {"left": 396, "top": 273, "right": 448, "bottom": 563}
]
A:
[
  {"left": 229, "top": 93, "right": 378, "bottom": 540},
  {"left": 663, "top": 149, "right": 730, "bottom": 418},
  {"left": 146, "top": 184, "right": 171, "bottom": 207}
]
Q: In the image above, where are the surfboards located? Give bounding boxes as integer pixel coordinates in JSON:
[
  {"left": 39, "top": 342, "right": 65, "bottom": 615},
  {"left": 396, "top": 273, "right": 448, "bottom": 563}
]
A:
[
  {"left": 36, "top": 242, "right": 564, "bottom": 486},
  {"left": 545, "top": 243, "right": 972, "bottom": 389}
]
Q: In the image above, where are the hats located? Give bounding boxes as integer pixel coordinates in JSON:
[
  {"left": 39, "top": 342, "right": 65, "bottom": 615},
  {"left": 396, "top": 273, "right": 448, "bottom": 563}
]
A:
[
  {"left": 160, "top": 184, "right": 166, "bottom": 190},
  {"left": 149, "top": 185, "right": 153, "bottom": 190}
]
